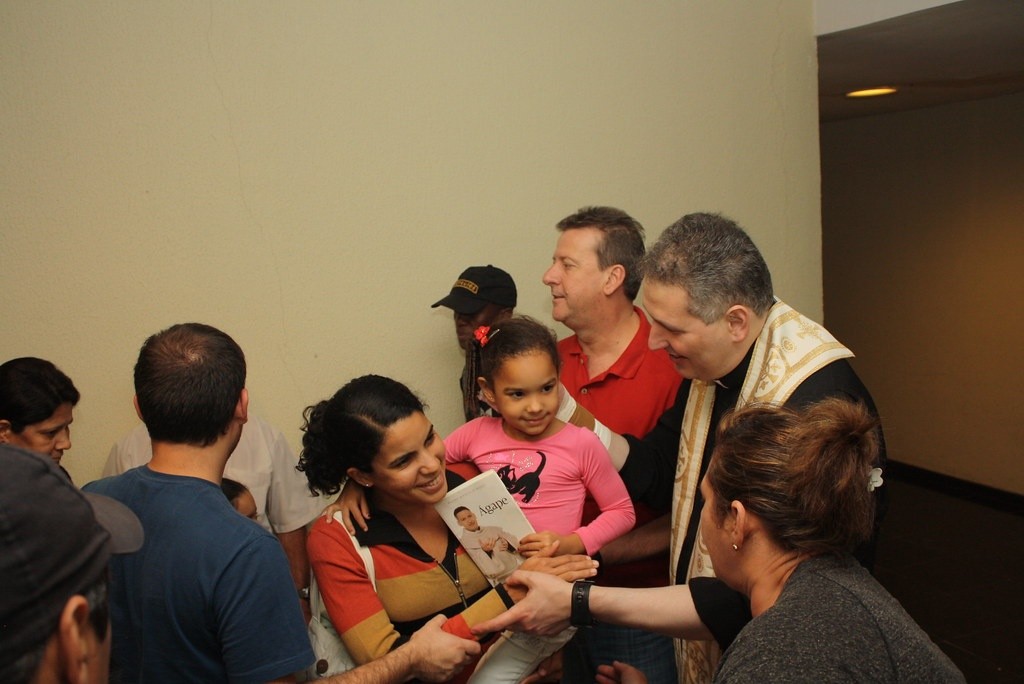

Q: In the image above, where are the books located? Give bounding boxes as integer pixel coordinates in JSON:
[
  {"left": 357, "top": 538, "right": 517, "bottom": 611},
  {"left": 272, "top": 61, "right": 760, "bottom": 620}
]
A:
[{"left": 434, "top": 470, "right": 537, "bottom": 588}]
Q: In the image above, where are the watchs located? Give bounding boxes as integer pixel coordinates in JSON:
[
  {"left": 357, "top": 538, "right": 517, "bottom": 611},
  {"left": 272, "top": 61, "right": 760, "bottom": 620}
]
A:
[{"left": 571, "top": 581, "right": 596, "bottom": 630}]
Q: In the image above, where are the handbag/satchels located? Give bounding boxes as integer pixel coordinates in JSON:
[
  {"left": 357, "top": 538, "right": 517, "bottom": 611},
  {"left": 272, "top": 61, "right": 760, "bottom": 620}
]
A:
[{"left": 294, "top": 512, "right": 376, "bottom": 682}]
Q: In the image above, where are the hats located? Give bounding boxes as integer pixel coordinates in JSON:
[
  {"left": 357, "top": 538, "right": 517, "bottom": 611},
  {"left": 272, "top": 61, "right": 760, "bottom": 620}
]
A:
[
  {"left": 0, "top": 444, "right": 145, "bottom": 664},
  {"left": 431, "top": 265, "right": 517, "bottom": 313}
]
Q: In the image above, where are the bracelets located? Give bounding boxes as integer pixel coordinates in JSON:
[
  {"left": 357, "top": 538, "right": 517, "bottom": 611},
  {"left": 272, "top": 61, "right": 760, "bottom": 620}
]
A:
[
  {"left": 299, "top": 587, "right": 309, "bottom": 598},
  {"left": 495, "top": 583, "right": 515, "bottom": 609}
]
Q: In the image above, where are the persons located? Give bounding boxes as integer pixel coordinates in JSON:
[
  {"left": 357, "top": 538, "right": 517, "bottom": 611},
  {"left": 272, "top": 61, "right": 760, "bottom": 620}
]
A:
[
  {"left": 294, "top": 375, "right": 600, "bottom": 684},
  {"left": 596, "top": 400, "right": 966, "bottom": 684},
  {"left": 1, "top": 322, "right": 480, "bottom": 683},
  {"left": 544, "top": 205, "right": 685, "bottom": 684},
  {"left": 430, "top": 264, "right": 517, "bottom": 422},
  {"left": 320, "top": 316, "right": 635, "bottom": 684},
  {"left": 470, "top": 213, "right": 888, "bottom": 684}
]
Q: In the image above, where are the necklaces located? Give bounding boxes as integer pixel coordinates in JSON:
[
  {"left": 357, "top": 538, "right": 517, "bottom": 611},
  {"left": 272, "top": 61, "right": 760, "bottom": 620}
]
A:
[{"left": 454, "top": 506, "right": 519, "bottom": 587}]
